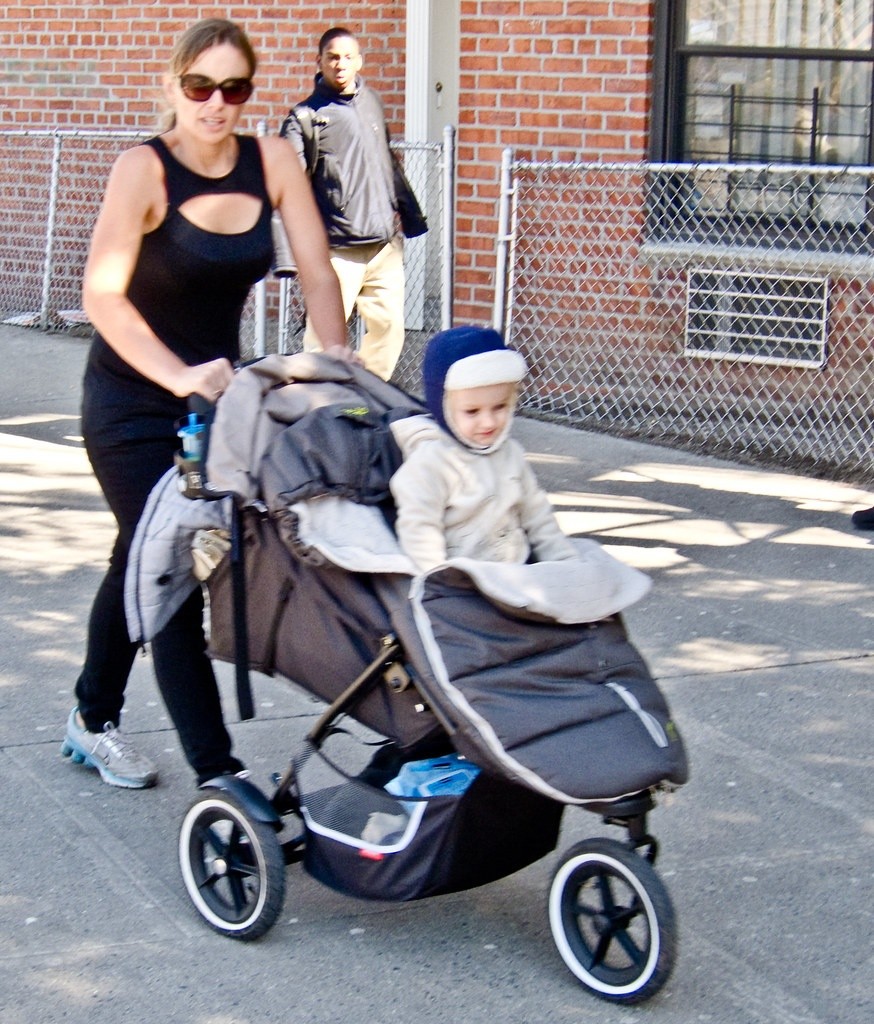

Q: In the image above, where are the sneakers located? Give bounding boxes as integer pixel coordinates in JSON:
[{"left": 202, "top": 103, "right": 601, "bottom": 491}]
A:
[{"left": 61, "top": 707, "right": 160, "bottom": 789}]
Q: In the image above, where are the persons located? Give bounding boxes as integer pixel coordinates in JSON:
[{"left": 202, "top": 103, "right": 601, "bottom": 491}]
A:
[
  {"left": 850, "top": 505, "right": 874, "bottom": 534},
  {"left": 274, "top": 27, "right": 429, "bottom": 384},
  {"left": 58, "top": 18, "right": 366, "bottom": 817},
  {"left": 390, "top": 323, "right": 574, "bottom": 579}
]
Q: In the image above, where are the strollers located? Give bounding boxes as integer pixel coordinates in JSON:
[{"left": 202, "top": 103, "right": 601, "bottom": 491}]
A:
[{"left": 174, "top": 353, "right": 680, "bottom": 1005}]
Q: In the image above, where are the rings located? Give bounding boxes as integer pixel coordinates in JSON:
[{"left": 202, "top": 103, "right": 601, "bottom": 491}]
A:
[{"left": 215, "top": 389, "right": 223, "bottom": 395}]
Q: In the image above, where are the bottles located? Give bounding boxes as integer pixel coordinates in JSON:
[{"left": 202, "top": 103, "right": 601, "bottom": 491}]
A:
[{"left": 177, "top": 413, "right": 207, "bottom": 489}]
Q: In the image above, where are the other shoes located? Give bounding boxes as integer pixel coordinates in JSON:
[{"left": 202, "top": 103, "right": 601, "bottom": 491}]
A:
[{"left": 852, "top": 506, "right": 874, "bottom": 531}]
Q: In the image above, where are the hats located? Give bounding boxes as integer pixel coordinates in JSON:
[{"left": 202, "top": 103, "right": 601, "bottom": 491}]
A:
[{"left": 423, "top": 325, "right": 527, "bottom": 455}]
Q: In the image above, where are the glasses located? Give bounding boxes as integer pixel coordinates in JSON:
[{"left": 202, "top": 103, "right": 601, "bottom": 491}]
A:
[{"left": 178, "top": 73, "right": 254, "bottom": 105}]
